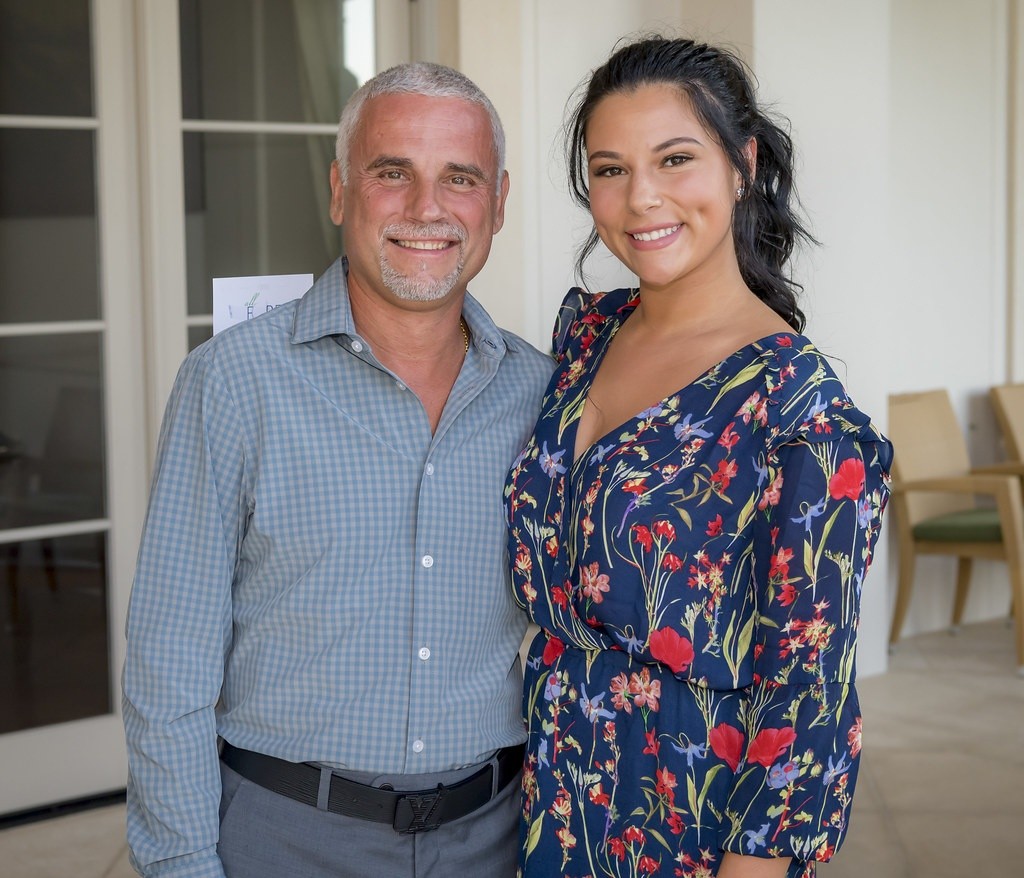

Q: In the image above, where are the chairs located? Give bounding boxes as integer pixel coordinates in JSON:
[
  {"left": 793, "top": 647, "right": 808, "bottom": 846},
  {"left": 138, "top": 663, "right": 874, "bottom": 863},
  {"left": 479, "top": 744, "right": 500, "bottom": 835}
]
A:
[
  {"left": 988, "top": 384, "right": 1024, "bottom": 459},
  {"left": 880, "top": 390, "right": 1024, "bottom": 666}
]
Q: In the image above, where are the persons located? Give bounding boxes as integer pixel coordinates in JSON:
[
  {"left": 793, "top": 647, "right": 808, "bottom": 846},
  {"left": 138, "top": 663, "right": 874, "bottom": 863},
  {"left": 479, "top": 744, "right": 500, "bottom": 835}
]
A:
[
  {"left": 502, "top": 15, "right": 895, "bottom": 877},
  {"left": 120, "top": 59, "right": 561, "bottom": 877}
]
